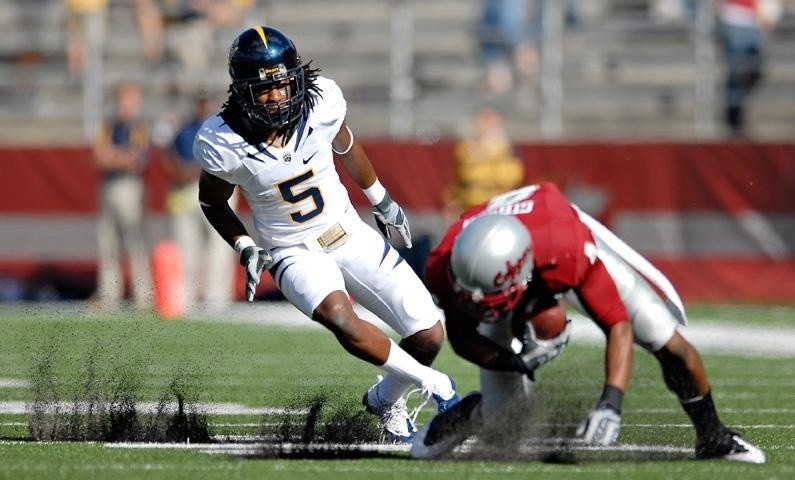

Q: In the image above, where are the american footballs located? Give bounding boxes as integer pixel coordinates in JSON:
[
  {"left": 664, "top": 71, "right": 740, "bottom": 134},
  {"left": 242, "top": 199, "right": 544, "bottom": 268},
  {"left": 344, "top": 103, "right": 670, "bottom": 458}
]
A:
[{"left": 510, "top": 295, "right": 565, "bottom": 343}]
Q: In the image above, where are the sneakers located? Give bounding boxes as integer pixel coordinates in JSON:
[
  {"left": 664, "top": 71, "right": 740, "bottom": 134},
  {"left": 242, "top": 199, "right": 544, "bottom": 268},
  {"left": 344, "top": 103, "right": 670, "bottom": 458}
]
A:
[
  {"left": 696, "top": 429, "right": 765, "bottom": 464},
  {"left": 363, "top": 385, "right": 415, "bottom": 442},
  {"left": 434, "top": 376, "right": 459, "bottom": 411},
  {"left": 411, "top": 392, "right": 482, "bottom": 459}
]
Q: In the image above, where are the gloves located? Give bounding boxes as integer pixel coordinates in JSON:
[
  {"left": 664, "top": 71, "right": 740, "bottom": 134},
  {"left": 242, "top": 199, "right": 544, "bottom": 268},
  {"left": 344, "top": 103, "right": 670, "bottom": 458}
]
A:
[
  {"left": 516, "top": 319, "right": 572, "bottom": 384},
  {"left": 362, "top": 177, "right": 413, "bottom": 248},
  {"left": 232, "top": 236, "right": 273, "bottom": 302},
  {"left": 575, "top": 385, "right": 623, "bottom": 446}
]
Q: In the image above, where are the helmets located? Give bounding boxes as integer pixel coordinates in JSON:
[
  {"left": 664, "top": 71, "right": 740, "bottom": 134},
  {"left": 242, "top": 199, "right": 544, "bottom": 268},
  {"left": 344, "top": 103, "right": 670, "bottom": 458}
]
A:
[
  {"left": 452, "top": 215, "right": 534, "bottom": 322},
  {"left": 228, "top": 25, "right": 305, "bottom": 128}
]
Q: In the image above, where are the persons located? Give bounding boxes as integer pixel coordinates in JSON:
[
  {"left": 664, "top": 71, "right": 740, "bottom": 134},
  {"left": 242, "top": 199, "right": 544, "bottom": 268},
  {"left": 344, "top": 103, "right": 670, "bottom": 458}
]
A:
[
  {"left": 474, "top": 0, "right": 542, "bottom": 93},
  {"left": 440, "top": 109, "right": 528, "bottom": 228},
  {"left": 716, "top": 1, "right": 770, "bottom": 134},
  {"left": 408, "top": 179, "right": 768, "bottom": 465},
  {"left": 84, "top": 83, "right": 159, "bottom": 312},
  {"left": 191, "top": 24, "right": 461, "bottom": 442},
  {"left": 161, "top": 82, "right": 240, "bottom": 316}
]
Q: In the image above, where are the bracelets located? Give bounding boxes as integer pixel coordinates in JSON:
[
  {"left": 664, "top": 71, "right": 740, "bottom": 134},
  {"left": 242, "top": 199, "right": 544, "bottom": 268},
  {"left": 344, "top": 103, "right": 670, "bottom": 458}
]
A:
[
  {"left": 233, "top": 236, "right": 258, "bottom": 255},
  {"left": 359, "top": 177, "right": 387, "bottom": 206},
  {"left": 595, "top": 385, "right": 625, "bottom": 416}
]
